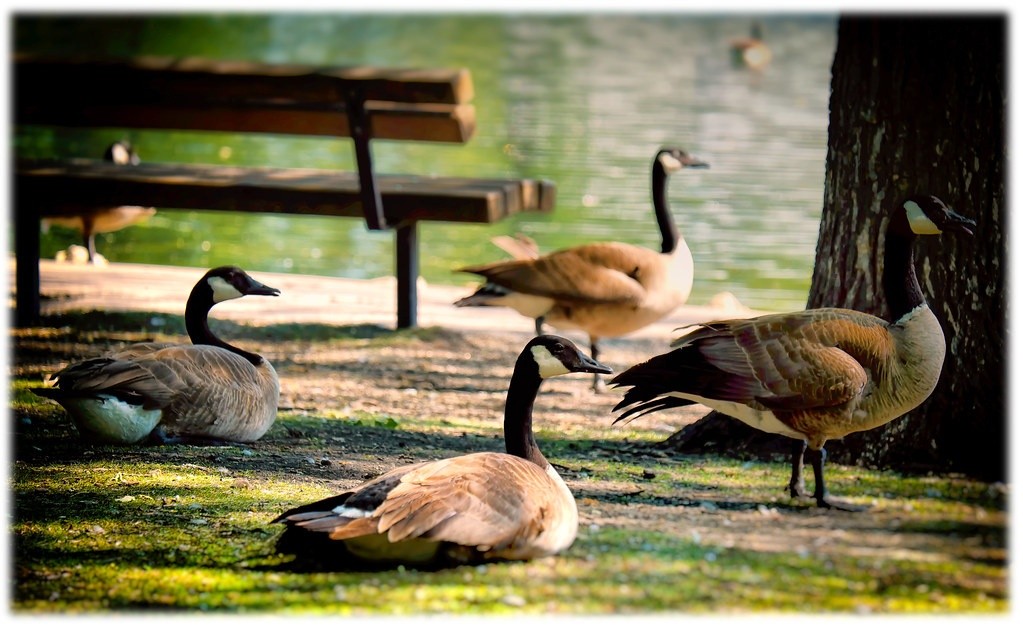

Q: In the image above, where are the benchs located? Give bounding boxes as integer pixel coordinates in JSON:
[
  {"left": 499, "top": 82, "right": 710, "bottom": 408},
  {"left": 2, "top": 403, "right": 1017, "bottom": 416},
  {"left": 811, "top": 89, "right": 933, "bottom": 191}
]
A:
[{"left": 12, "top": 51, "right": 557, "bottom": 331}]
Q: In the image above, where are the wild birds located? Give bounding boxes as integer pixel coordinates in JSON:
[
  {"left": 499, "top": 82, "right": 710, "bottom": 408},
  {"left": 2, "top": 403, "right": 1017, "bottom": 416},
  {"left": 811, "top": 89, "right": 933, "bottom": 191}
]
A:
[
  {"left": 450, "top": 146, "right": 711, "bottom": 396},
  {"left": 267, "top": 334, "right": 613, "bottom": 569},
  {"left": 728, "top": 18, "right": 774, "bottom": 71},
  {"left": 605, "top": 192, "right": 977, "bottom": 514},
  {"left": 28, "top": 263, "right": 281, "bottom": 447},
  {"left": 41, "top": 140, "right": 158, "bottom": 265}
]
